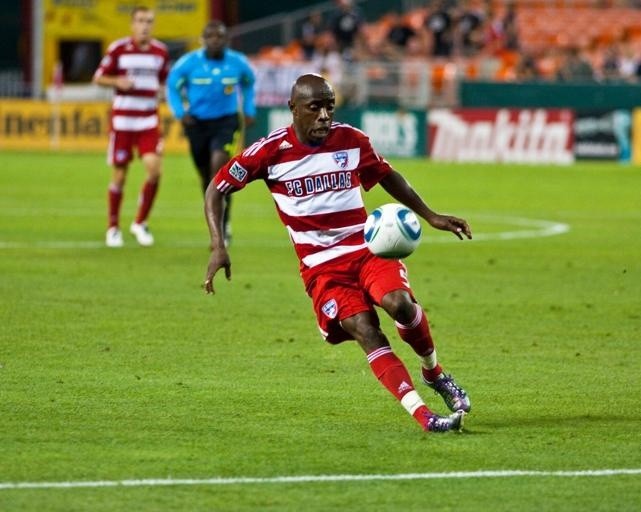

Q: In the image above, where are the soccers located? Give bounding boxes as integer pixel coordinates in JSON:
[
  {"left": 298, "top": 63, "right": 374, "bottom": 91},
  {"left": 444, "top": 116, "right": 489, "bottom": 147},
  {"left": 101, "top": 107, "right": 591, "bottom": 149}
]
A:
[{"left": 363, "top": 203, "right": 421, "bottom": 259}]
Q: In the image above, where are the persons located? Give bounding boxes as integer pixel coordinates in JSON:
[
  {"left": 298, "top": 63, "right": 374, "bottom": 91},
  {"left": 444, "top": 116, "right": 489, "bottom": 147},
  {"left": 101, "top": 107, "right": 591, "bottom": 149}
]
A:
[
  {"left": 294, "top": 0, "right": 640, "bottom": 83},
  {"left": 163, "top": 20, "right": 260, "bottom": 251},
  {"left": 88, "top": 3, "right": 174, "bottom": 250},
  {"left": 201, "top": 72, "right": 475, "bottom": 434}
]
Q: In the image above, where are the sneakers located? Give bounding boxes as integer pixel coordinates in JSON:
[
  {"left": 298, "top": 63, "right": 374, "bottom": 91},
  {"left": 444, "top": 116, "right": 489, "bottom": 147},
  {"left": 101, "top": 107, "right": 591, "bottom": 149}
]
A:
[
  {"left": 426, "top": 409, "right": 465, "bottom": 434},
  {"left": 421, "top": 367, "right": 471, "bottom": 414},
  {"left": 129, "top": 222, "right": 155, "bottom": 247},
  {"left": 105, "top": 226, "right": 125, "bottom": 250}
]
